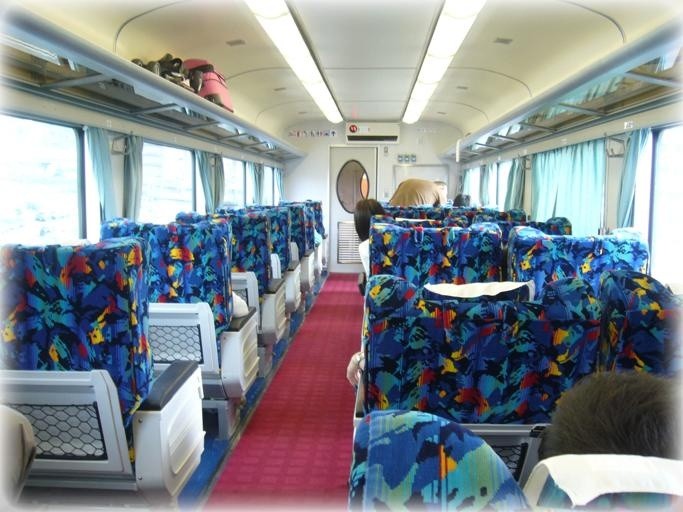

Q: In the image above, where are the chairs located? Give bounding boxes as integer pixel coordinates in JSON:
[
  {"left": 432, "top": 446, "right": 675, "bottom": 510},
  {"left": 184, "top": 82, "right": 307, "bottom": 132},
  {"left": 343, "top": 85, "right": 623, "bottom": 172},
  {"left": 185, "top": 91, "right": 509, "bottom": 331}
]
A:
[
  {"left": 538, "top": 271, "right": 682, "bottom": 488},
  {"left": 347, "top": 407, "right": 534, "bottom": 512},
  {"left": 0, "top": 240, "right": 204, "bottom": 512},
  {"left": 525, "top": 409, "right": 683, "bottom": 512},
  {"left": 367, "top": 200, "right": 649, "bottom": 291},
  {"left": 352, "top": 274, "right": 538, "bottom": 494}
]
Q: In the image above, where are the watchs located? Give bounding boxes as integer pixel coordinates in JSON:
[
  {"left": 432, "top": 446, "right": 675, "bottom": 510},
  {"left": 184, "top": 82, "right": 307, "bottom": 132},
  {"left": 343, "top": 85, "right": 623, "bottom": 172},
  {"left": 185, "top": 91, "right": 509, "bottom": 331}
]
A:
[{"left": 354, "top": 351, "right": 365, "bottom": 368}]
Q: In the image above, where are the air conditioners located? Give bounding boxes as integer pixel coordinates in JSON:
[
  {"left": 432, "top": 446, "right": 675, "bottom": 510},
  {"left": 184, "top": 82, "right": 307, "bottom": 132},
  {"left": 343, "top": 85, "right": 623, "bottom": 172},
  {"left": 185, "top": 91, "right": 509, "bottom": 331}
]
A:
[{"left": 345, "top": 123, "right": 400, "bottom": 144}]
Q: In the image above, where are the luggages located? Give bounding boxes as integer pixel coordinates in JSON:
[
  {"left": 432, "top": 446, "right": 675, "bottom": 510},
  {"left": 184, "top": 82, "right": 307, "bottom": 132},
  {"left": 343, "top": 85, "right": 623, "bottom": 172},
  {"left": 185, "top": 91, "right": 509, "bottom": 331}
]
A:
[{"left": 183, "top": 58, "right": 234, "bottom": 113}]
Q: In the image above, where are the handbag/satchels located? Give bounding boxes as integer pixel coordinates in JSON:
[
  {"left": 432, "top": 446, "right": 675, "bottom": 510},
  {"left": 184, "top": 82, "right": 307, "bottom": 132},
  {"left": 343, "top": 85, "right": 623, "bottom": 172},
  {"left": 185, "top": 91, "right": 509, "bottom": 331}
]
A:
[{"left": 160, "top": 54, "right": 182, "bottom": 75}]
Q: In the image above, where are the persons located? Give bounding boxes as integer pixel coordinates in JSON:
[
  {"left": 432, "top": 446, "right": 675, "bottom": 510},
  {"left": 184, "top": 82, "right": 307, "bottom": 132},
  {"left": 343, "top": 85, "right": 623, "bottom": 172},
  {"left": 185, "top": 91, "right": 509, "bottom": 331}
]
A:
[
  {"left": 315, "top": 229, "right": 322, "bottom": 248},
  {"left": 346, "top": 351, "right": 365, "bottom": 387},
  {"left": 354, "top": 199, "right": 384, "bottom": 276},
  {"left": 0, "top": 403, "right": 38, "bottom": 510},
  {"left": 453, "top": 193, "right": 472, "bottom": 207},
  {"left": 539, "top": 368, "right": 683, "bottom": 462},
  {"left": 230, "top": 289, "right": 248, "bottom": 319},
  {"left": 387, "top": 178, "right": 446, "bottom": 208}
]
